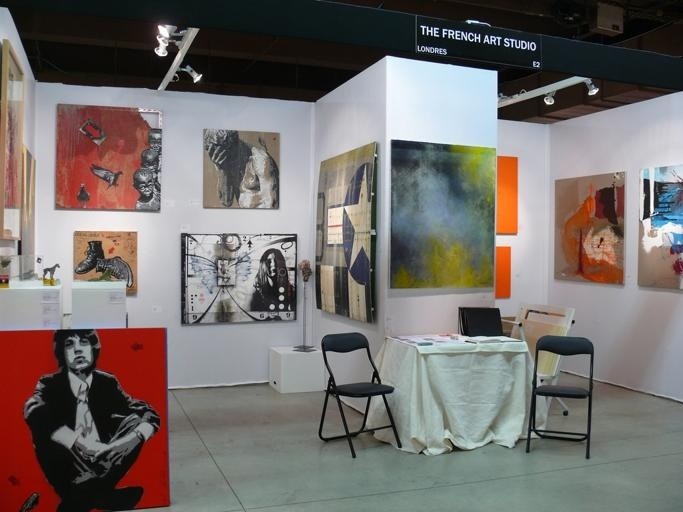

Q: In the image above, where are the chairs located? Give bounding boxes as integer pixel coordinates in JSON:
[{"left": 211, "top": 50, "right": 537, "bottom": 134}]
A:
[
  {"left": 499, "top": 303, "right": 578, "bottom": 422},
  {"left": 456, "top": 304, "right": 505, "bottom": 334},
  {"left": 522, "top": 335, "right": 594, "bottom": 457},
  {"left": 315, "top": 330, "right": 401, "bottom": 457}
]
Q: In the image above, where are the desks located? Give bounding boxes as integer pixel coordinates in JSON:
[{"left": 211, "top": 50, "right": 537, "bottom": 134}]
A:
[{"left": 363, "top": 334, "right": 548, "bottom": 456}]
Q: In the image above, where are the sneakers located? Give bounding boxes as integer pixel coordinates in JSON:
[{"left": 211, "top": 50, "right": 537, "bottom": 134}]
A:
[
  {"left": 75, "top": 240, "right": 106, "bottom": 275},
  {"left": 94, "top": 255, "right": 133, "bottom": 288},
  {"left": 95, "top": 485, "right": 143, "bottom": 511}
]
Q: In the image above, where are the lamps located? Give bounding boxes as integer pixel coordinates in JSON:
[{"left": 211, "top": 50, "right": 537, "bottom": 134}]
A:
[
  {"left": 498, "top": 76, "right": 602, "bottom": 112},
  {"left": 148, "top": 24, "right": 207, "bottom": 94}
]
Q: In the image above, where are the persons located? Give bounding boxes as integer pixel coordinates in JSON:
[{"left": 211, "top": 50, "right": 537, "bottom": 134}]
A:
[
  {"left": 249, "top": 248, "right": 295, "bottom": 311},
  {"left": 147, "top": 129, "right": 161, "bottom": 155},
  {"left": 139, "top": 149, "right": 160, "bottom": 191},
  {"left": 202, "top": 129, "right": 278, "bottom": 210},
  {"left": 130, "top": 168, "right": 160, "bottom": 211},
  {"left": 22, "top": 329, "right": 161, "bottom": 512}
]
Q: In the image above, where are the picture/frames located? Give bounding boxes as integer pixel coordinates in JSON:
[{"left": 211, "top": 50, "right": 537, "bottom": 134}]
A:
[{"left": 0, "top": 36, "right": 27, "bottom": 245}]
